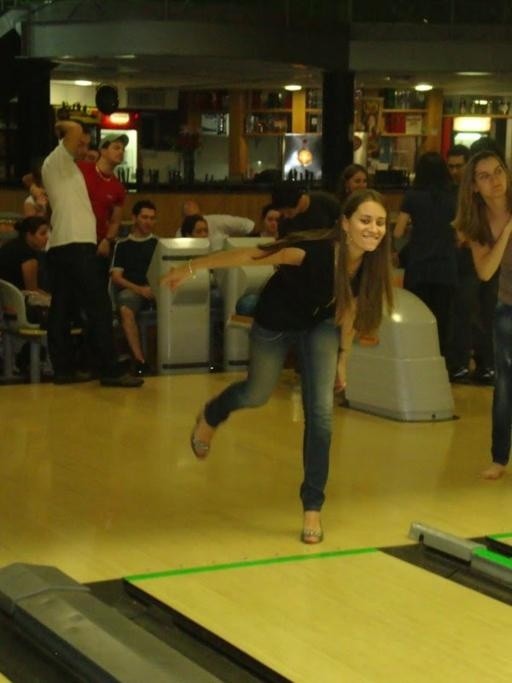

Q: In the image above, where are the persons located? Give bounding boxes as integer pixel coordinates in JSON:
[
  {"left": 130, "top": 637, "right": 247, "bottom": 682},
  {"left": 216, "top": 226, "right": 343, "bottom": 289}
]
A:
[
  {"left": 1, "top": 118, "right": 511, "bottom": 479},
  {"left": 160, "top": 188, "right": 394, "bottom": 543}
]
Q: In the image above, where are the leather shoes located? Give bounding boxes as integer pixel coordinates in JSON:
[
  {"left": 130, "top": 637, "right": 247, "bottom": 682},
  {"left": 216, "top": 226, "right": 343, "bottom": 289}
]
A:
[
  {"left": 55, "top": 374, "right": 90, "bottom": 384},
  {"left": 474, "top": 368, "right": 494, "bottom": 384},
  {"left": 451, "top": 368, "right": 473, "bottom": 383},
  {"left": 100, "top": 373, "right": 145, "bottom": 388}
]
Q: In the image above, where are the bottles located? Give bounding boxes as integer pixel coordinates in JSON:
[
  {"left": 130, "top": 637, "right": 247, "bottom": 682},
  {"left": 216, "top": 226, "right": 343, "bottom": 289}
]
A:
[{"left": 217, "top": 113, "right": 226, "bottom": 136}]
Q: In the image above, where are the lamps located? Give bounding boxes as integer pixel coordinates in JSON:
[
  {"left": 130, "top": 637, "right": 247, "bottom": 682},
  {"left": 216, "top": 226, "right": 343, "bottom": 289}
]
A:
[
  {"left": 108, "top": 112, "right": 132, "bottom": 127},
  {"left": 297, "top": 138, "right": 313, "bottom": 167}
]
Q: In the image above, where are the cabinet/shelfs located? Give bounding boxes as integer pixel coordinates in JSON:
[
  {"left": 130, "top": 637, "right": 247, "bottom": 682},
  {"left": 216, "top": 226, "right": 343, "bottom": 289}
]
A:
[{"left": 174, "top": 84, "right": 445, "bottom": 184}]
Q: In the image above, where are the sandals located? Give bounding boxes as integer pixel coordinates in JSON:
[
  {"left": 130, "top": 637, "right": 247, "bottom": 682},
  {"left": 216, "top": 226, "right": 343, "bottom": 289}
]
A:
[
  {"left": 301, "top": 521, "right": 323, "bottom": 545},
  {"left": 191, "top": 406, "right": 211, "bottom": 458}
]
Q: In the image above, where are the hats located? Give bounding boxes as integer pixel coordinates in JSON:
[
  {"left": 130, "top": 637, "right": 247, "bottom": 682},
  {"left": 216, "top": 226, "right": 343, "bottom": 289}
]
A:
[{"left": 99, "top": 133, "right": 129, "bottom": 149}]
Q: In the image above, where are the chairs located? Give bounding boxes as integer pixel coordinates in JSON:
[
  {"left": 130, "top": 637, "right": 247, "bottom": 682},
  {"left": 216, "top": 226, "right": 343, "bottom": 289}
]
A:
[
  {"left": 106, "top": 276, "right": 159, "bottom": 371},
  {"left": 0, "top": 280, "right": 81, "bottom": 383}
]
